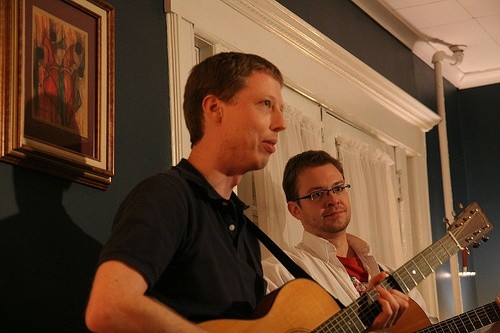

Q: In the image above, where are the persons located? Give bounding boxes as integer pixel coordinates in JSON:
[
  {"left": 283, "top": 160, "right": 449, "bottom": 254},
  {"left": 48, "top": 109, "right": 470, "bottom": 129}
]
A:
[
  {"left": 261, "top": 149, "right": 500, "bottom": 332},
  {"left": 85, "top": 50, "right": 410, "bottom": 333}
]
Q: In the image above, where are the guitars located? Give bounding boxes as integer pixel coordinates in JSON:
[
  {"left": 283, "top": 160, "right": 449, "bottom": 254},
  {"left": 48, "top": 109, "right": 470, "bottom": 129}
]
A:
[
  {"left": 195, "top": 201, "right": 495, "bottom": 333},
  {"left": 365, "top": 293, "right": 500, "bottom": 333}
]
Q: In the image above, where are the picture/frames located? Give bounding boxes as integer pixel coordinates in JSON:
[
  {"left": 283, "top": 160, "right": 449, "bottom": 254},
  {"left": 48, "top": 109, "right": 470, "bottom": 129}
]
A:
[{"left": 1, "top": 0, "right": 114, "bottom": 189}]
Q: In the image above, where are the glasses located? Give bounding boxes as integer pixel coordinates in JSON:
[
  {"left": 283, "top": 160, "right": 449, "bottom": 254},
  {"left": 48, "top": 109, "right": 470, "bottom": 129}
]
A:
[{"left": 294, "top": 183, "right": 354, "bottom": 203}]
